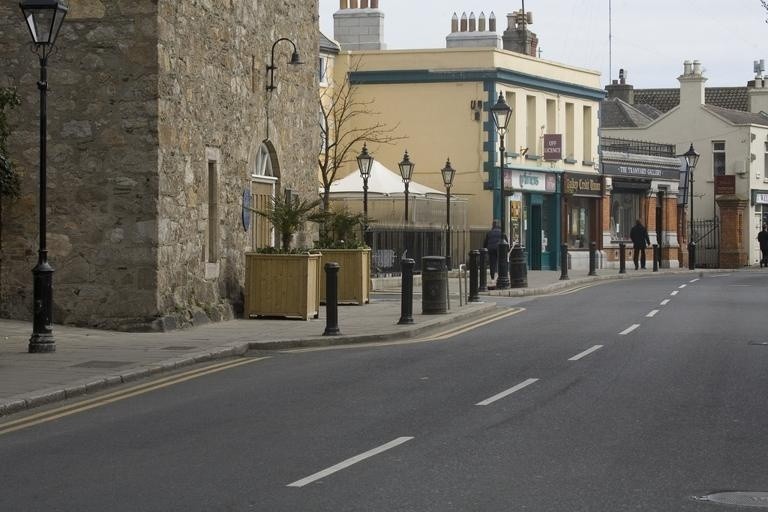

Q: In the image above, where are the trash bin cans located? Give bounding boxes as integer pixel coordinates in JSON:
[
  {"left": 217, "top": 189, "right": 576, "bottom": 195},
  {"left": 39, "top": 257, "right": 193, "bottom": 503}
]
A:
[
  {"left": 509, "top": 244, "right": 527, "bottom": 288},
  {"left": 421, "top": 256, "right": 447, "bottom": 314}
]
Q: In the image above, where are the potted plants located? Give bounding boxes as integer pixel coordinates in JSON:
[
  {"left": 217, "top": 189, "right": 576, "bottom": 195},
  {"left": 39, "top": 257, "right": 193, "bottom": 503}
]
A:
[
  {"left": 309, "top": 209, "right": 376, "bottom": 306},
  {"left": 242, "top": 188, "right": 338, "bottom": 321}
]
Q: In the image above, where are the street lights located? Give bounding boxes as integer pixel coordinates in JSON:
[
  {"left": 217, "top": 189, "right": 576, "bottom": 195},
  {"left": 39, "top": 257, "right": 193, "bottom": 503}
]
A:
[
  {"left": 398, "top": 148, "right": 415, "bottom": 274},
  {"left": 356, "top": 141, "right": 374, "bottom": 276},
  {"left": 18, "top": 1, "right": 70, "bottom": 353},
  {"left": 683, "top": 142, "right": 700, "bottom": 270},
  {"left": 440, "top": 156, "right": 457, "bottom": 271},
  {"left": 489, "top": 89, "right": 513, "bottom": 290}
]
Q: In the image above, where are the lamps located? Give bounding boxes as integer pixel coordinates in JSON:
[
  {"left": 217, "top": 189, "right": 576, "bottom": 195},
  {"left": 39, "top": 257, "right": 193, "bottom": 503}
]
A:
[{"left": 266, "top": 37, "right": 303, "bottom": 91}]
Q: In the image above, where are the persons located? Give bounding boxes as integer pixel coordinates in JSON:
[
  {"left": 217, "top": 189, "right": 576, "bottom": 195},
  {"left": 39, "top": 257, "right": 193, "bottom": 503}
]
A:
[
  {"left": 483, "top": 218, "right": 510, "bottom": 283},
  {"left": 628, "top": 219, "right": 651, "bottom": 270},
  {"left": 756, "top": 223, "right": 768, "bottom": 269}
]
